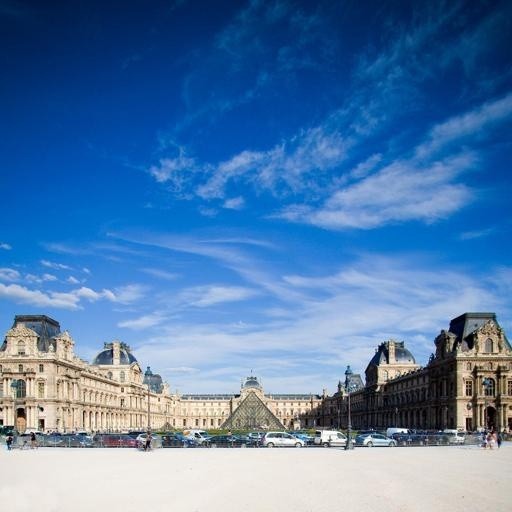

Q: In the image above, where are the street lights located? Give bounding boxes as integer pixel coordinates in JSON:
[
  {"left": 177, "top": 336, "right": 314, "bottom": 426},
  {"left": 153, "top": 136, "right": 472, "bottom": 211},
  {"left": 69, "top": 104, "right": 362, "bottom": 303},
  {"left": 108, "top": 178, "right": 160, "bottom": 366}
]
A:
[
  {"left": 394, "top": 406, "right": 398, "bottom": 427},
  {"left": 344, "top": 364, "right": 355, "bottom": 451},
  {"left": 143, "top": 364, "right": 154, "bottom": 450},
  {"left": 481, "top": 376, "right": 492, "bottom": 432},
  {"left": 9, "top": 378, "right": 19, "bottom": 430},
  {"left": 35, "top": 403, "right": 40, "bottom": 431}
]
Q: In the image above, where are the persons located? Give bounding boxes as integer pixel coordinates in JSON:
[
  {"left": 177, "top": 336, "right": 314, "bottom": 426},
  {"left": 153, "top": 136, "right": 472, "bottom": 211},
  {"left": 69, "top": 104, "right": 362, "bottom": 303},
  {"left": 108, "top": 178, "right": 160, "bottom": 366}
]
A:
[
  {"left": 29, "top": 432, "right": 37, "bottom": 449},
  {"left": 144, "top": 432, "right": 152, "bottom": 451},
  {"left": 480, "top": 425, "right": 512, "bottom": 450},
  {"left": 6, "top": 436, "right": 13, "bottom": 450}
]
